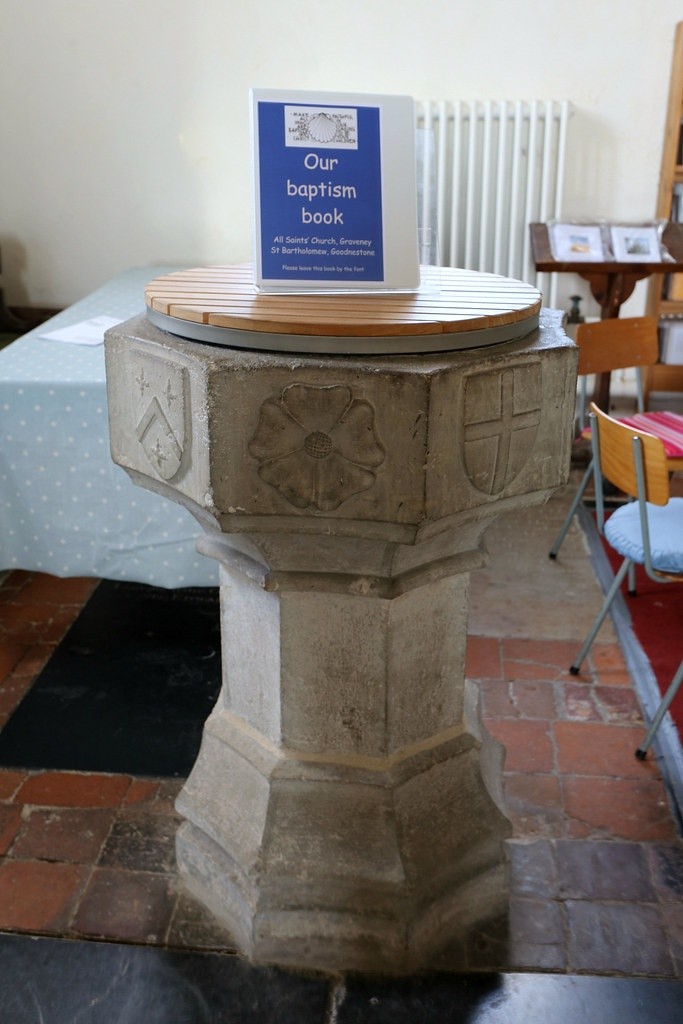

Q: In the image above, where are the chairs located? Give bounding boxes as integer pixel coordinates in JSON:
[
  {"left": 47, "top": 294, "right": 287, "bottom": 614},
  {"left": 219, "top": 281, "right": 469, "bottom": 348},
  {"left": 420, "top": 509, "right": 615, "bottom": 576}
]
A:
[
  {"left": 568, "top": 401, "right": 683, "bottom": 760},
  {"left": 547, "top": 315, "right": 658, "bottom": 597}
]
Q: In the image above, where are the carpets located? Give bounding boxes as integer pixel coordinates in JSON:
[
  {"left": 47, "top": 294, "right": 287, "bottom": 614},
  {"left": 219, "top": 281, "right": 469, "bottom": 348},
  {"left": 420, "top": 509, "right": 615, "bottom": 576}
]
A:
[{"left": 0, "top": 578, "right": 222, "bottom": 780}]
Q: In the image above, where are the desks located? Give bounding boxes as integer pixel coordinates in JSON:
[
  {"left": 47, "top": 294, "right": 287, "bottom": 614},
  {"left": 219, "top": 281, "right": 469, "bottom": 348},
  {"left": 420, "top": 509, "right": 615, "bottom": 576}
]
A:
[
  {"left": 99, "top": 267, "right": 577, "bottom": 985},
  {"left": 0, "top": 278, "right": 218, "bottom": 588}
]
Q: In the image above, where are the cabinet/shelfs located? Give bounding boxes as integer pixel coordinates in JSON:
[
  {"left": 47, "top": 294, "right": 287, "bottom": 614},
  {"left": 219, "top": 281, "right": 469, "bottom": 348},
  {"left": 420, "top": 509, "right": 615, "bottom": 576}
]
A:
[{"left": 634, "top": 20, "right": 683, "bottom": 414}]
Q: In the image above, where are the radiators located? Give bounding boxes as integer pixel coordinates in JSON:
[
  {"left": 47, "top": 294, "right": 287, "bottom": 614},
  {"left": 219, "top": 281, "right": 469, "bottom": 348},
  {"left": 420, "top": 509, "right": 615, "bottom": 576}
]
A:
[{"left": 412, "top": 97, "right": 572, "bottom": 281}]
